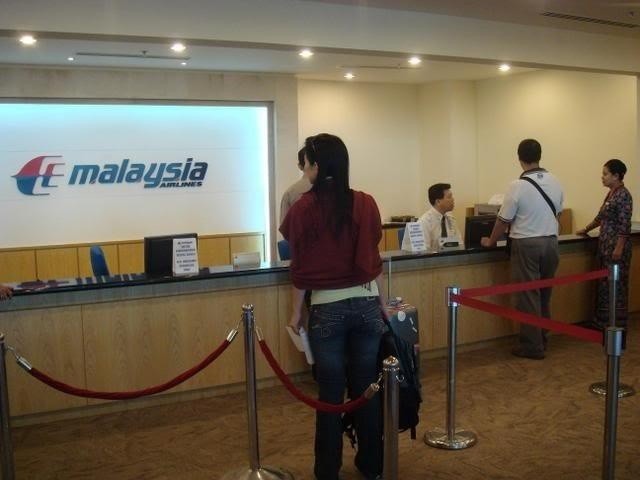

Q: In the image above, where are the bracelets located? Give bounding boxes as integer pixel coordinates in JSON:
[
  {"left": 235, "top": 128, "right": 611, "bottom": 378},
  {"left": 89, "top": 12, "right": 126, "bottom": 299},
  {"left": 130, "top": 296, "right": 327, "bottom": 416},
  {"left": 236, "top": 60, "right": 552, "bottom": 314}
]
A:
[{"left": 584, "top": 227, "right": 590, "bottom": 232}]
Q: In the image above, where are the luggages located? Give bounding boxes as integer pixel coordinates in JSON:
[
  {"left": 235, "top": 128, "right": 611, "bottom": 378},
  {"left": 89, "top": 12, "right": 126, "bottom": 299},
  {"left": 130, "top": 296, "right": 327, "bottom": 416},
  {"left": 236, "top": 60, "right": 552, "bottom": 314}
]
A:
[{"left": 377, "top": 254, "right": 420, "bottom": 370}]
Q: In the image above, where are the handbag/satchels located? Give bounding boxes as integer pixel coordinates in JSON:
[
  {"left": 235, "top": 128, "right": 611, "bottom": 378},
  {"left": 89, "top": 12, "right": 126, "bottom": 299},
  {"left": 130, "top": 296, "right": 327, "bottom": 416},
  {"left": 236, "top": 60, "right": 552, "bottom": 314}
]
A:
[{"left": 378, "top": 327, "right": 422, "bottom": 440}]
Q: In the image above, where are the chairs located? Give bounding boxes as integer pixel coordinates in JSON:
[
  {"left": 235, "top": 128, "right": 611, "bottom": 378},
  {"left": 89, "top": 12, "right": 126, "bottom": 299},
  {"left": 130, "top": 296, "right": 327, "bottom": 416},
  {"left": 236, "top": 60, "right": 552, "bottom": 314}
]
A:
[{"left": 91, "top": 246, "right": 108, "bottom": 275}]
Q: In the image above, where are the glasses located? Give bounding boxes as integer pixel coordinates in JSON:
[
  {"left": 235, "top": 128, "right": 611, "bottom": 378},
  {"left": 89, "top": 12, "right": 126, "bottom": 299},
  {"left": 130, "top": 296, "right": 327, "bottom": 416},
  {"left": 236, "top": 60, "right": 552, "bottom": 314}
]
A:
[{"left": 305, "top": 136, "right": 316, "bottom": 152}]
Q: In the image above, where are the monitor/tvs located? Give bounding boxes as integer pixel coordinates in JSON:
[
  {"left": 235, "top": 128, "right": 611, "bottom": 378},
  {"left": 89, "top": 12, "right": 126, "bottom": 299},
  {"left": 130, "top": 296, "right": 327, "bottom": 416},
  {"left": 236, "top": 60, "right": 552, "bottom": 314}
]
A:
[
  {"left": 464, "top": 215, "right": 505, "bottom": 249},
  {"left": 143, "top": 233, "right": 199, "bottom": 279}
]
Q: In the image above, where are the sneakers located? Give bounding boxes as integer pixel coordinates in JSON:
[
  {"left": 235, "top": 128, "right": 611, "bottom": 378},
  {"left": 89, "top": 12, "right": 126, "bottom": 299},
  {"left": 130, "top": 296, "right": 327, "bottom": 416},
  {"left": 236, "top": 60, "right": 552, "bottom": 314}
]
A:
[{"left": 512, "top": 340, "right": 547, "bottom": 359}]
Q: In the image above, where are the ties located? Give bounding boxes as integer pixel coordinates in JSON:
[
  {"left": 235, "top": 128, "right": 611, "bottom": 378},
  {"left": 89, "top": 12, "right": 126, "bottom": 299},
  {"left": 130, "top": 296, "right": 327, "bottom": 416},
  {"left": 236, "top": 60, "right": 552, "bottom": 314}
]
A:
[{"left": 441, "top": 215, "right": 447, "bottom": 237}]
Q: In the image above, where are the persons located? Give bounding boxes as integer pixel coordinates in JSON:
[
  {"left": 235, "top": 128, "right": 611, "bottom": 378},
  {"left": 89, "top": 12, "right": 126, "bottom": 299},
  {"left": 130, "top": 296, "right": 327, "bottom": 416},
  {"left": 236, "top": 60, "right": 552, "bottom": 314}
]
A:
[
  {"left": 278, "top": 132, "right": 383, "bottom": 480},
  {"left": 279, "top": 145, "right": 312, "bottom": 229},
  {"left": 415, "top": 184, "right": 464, "bottom": 249},
  {"left": 574, "top": 159, "right": 634, "bottom": 352},
  {"left": 481, "top": 138, "right": 564, "bottom": 359}
]
